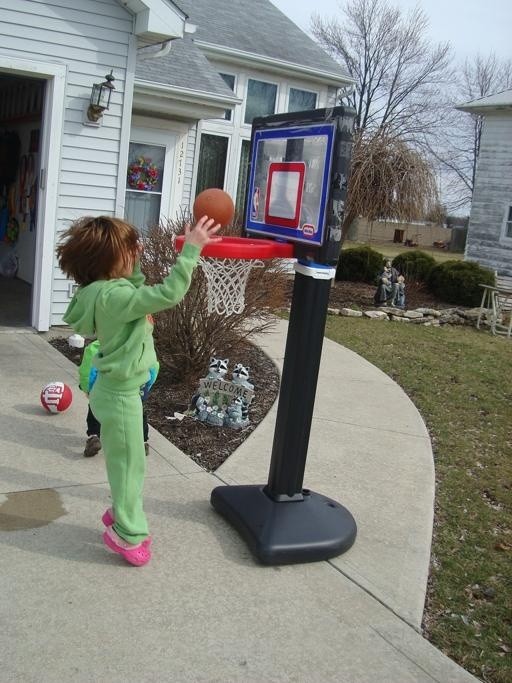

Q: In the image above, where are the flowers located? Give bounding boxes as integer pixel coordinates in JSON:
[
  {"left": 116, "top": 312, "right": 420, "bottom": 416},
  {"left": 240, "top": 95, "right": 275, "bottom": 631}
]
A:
[{"left": 127, "top": 156, "right": 160, "bottom": 191}]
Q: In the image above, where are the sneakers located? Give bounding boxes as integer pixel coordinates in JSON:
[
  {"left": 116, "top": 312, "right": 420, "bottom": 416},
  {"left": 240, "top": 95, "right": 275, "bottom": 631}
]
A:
[
  {"left": 84, "top": 436, "right": 102, "bottom": 458},
  {"left": 145, "top": 443, "right": 150, "bottom": 456},
  {"left": 101, "top": 508, "right": 153, "bottom": 566}
]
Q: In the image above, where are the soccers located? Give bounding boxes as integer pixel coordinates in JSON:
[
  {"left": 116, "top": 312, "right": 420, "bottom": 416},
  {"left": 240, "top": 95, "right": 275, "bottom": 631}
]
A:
[{"left": 41, "top": 382, "right": 72, "bottom": 414}]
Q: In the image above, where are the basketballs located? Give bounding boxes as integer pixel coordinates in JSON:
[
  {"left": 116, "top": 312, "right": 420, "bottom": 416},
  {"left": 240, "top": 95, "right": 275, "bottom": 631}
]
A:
[{"left": 193, "top": 188, "right": 234, "bottom": 230}]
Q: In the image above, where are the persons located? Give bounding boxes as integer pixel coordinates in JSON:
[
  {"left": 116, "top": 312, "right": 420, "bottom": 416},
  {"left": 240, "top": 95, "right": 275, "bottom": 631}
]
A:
[
  {"left": 77, "top": 338, "right": 159, "bottom": 458},
  {"left": 53, "top": 215, "right": 222, "bottom": 565},
  {"left": 373, "top": 258, "right": 406, "bottom": 310}
]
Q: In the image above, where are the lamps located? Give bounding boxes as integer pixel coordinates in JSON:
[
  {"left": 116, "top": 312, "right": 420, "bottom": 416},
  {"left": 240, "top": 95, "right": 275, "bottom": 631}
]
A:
[{"left": 87, "top": 68, "right": 116, "bottom": 122}]
